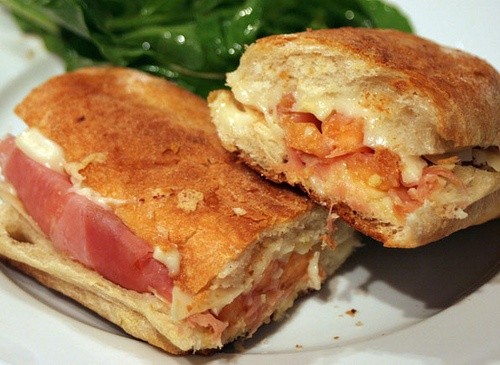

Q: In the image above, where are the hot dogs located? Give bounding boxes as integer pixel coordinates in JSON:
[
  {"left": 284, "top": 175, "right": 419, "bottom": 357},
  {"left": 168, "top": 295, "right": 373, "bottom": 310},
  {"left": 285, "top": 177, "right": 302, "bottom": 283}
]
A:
[
  {"left": 0, "top": 65, "right": 365, "bottom": 356},
  {"left": 206, "top": 26, "right": 500, "bottom": 250}
]
